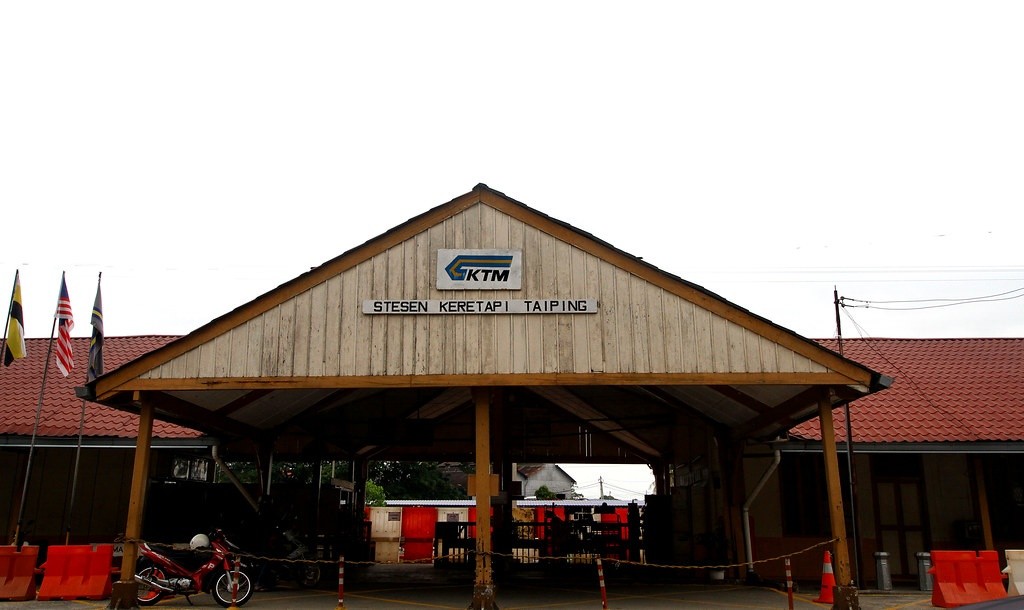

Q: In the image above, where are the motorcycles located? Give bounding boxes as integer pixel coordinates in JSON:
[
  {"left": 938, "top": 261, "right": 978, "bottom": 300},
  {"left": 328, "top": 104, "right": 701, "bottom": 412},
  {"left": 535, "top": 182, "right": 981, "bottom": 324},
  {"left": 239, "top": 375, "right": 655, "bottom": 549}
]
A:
[
  {"left": 216, "top": 528, "right": 323, "bottom": 591},
  {"left": 135, "top": 529, "right": 253, "bottom": 608}
]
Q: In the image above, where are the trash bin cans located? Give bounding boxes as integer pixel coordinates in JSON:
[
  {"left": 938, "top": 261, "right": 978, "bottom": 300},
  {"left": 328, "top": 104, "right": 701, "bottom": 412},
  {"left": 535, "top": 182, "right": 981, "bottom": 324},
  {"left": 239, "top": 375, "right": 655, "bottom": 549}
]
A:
[
  {"left": 876, "top": 550, "right": 893, "bottom": 591},
  {"left": 915, "top": 551, "right": 935, "bottom": 591}
]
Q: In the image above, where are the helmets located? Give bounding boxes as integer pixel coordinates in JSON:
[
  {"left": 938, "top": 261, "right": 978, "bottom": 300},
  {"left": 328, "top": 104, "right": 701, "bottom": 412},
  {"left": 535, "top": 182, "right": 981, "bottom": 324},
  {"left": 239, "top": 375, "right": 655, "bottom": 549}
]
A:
[{"left": 189, "top": 533, "right": 211, "bottom": 554}]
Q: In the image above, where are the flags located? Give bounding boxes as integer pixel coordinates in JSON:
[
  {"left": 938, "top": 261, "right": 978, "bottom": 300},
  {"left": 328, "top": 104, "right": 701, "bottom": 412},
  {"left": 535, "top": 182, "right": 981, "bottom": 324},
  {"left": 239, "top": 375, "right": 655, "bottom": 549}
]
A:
[
  {"left": 88, "top": 283, "right": 104, "bottom": 381},
  {"left": 4, "top": 273, "right": 27, "bottom": 367},
  {"left": 55, "top": 278, "right": 74, "bottom": 377}
]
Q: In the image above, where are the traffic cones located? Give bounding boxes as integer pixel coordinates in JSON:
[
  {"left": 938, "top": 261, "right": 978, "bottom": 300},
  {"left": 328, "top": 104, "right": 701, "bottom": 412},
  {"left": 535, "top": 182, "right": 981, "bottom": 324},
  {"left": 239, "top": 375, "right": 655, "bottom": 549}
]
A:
[{"left": 810, "top": 552, "right": 837, "bottom": 604}]
